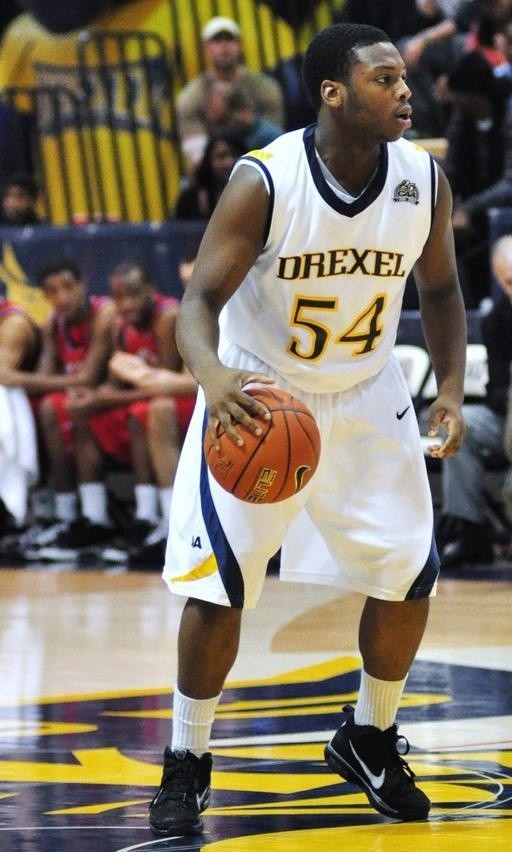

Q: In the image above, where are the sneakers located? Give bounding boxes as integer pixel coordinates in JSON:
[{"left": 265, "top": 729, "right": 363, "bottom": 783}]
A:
[
  {"left": 0, "top": 515, "right": 167, "bottom": 569},
  {"left": 324, "top": 705, "right": 430, "bottom": 822},
  {"left": 149, "top": 747, "right": 213, "bottom": 837}
]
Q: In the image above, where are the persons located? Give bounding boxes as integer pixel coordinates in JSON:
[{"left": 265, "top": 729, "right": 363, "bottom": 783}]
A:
[{"left": 149, "top": 23, "right": 467, "bottom": 837}]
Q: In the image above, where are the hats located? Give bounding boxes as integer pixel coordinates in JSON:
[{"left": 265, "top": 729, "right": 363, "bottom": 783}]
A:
[{"left": 201, "top": 17, "right": 239, "bottom": 42}]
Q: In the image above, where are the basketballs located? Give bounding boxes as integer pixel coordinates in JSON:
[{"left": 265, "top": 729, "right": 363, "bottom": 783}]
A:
[{"left": 203, "top": 385, "right": 323, "bottom": 505}]
